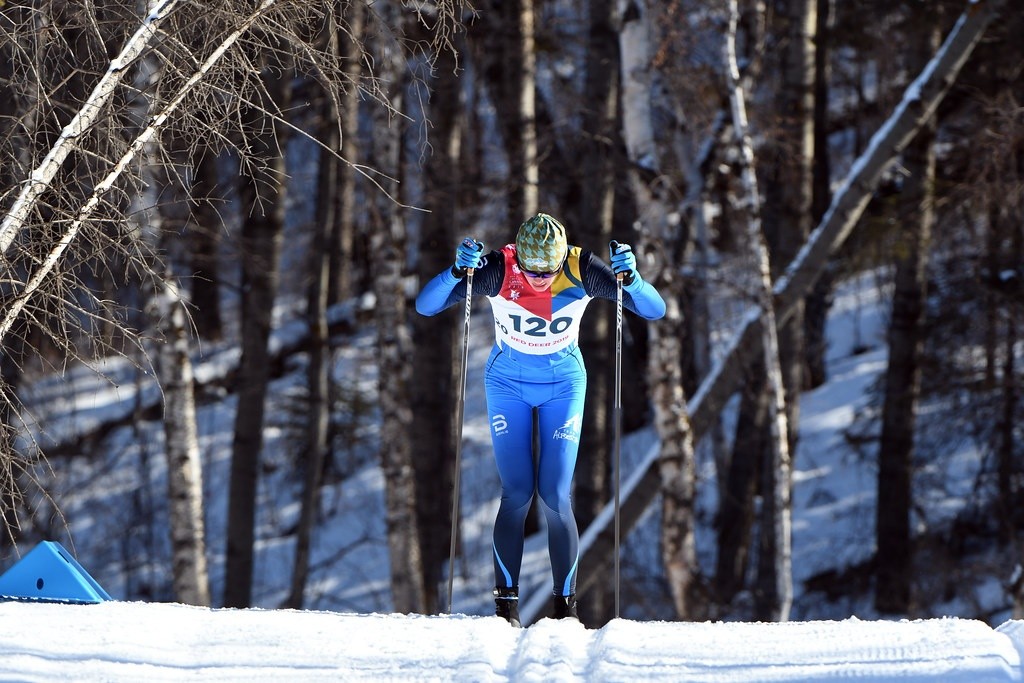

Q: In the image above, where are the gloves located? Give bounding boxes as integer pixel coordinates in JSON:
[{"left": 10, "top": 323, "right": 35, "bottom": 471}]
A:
[
  {"left": 454, "top": 236, "right": 488, "bottom": 272},
  {"left": 608, "top": 240, "right": 637, "bottom": 286}
]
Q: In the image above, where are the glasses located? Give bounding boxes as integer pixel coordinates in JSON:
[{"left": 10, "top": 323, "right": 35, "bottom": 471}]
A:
[{"left": 515, "top": 251, "right": 561, "bottom": 278}]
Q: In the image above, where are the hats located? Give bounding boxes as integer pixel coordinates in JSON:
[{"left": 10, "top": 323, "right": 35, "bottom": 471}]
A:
[{"left": 514, "top": 212, "right": 568, "bottom": 275}]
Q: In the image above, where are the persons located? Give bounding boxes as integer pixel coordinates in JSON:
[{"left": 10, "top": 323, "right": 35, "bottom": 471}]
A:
[{"left": 417, "top": 212, "right": 667, "bottom": 628}]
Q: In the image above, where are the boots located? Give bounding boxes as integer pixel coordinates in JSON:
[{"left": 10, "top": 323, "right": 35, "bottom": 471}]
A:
[
  {"left": 553, "top": 594, "right": 579, "bottom": 621},
  {"left": 493, "top": 584, "right": 521, "bottom": 628}
]
